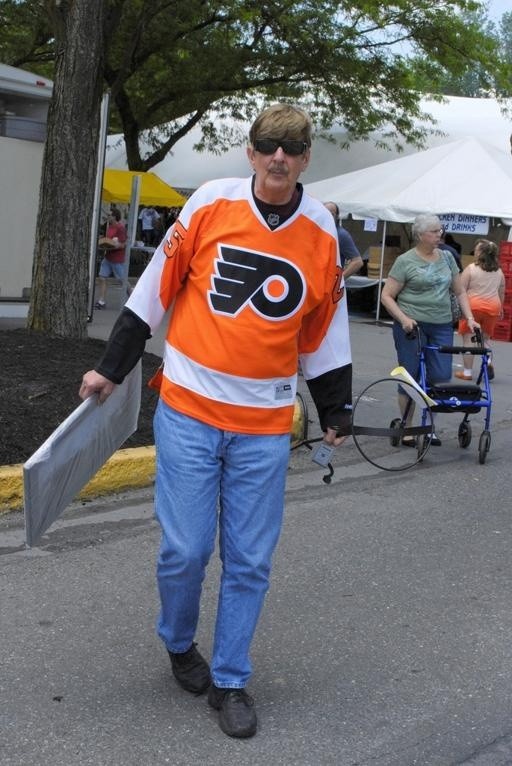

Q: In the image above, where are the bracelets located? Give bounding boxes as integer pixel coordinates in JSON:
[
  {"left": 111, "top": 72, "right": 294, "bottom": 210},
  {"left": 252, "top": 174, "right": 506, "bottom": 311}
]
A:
[{"left": 466, "top": 317, "right": 474, "bottom": 321}]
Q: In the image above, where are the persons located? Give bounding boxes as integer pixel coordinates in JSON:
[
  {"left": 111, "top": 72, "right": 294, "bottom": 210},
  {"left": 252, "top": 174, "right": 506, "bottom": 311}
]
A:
[
  {"left": 454, "top": 238, "right": 506, "bottom": 380},
  {"left": 78, "top": 104, "right": 352, "bottom": 739},
  {"left": 380, "top": 213, "right": 483, "bottom": 448},
  {"left": 322, "top": 200, "right": 363, "bottom": 283},
  {"left": 94, "top": 207, "right": 135, "bottom": 310},
  {"left": 100, "top": 202, "right": 183, "bottom": 250},
  {"left": 443, "top": 229, "right": 462, "bottom": 253},
  {"left": 441, "top": 224, "right": 466, "bottom": 332}
]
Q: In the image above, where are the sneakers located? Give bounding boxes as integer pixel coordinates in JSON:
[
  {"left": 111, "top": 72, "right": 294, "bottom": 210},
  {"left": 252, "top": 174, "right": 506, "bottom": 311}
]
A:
[
  {"left": 454, "top": 369, "right": 473, "bottom": 380},
  {"left": 205, "top": 683, "right": 257, "bottom": 739},
  {"left": 166, "top": 640, "right": 212, "bottom": 695},
  {"left": 486, "top": 364, "right": 495, "bottom": 381},
  {"left": 93, "top": 301, "right": 108, "bottom": 309}
]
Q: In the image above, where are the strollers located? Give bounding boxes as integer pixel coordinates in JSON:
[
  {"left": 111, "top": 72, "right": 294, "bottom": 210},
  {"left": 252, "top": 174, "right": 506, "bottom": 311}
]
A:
[{"left": 388, "top": 315, "right": 495, "bottom": 464}]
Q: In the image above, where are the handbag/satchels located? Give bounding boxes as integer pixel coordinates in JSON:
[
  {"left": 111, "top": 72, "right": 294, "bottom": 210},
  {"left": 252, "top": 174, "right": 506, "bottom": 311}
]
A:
[{"left": 450, "top": 290, "right": 462, "bottom": 323}]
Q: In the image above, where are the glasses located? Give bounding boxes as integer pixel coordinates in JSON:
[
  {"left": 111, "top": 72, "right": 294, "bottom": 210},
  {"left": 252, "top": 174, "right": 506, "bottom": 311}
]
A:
[{"left": 249, "top": 134, "right": 310, "bottom": 157}]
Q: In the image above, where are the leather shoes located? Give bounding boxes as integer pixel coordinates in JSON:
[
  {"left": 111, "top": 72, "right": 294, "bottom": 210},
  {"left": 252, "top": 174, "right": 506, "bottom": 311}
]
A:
[
  {"left": 425, "top": 432, "right": 442, "bottom": 447},
  {"left": 402, "top": 433, "right": 416, "bottom": 447}
]
